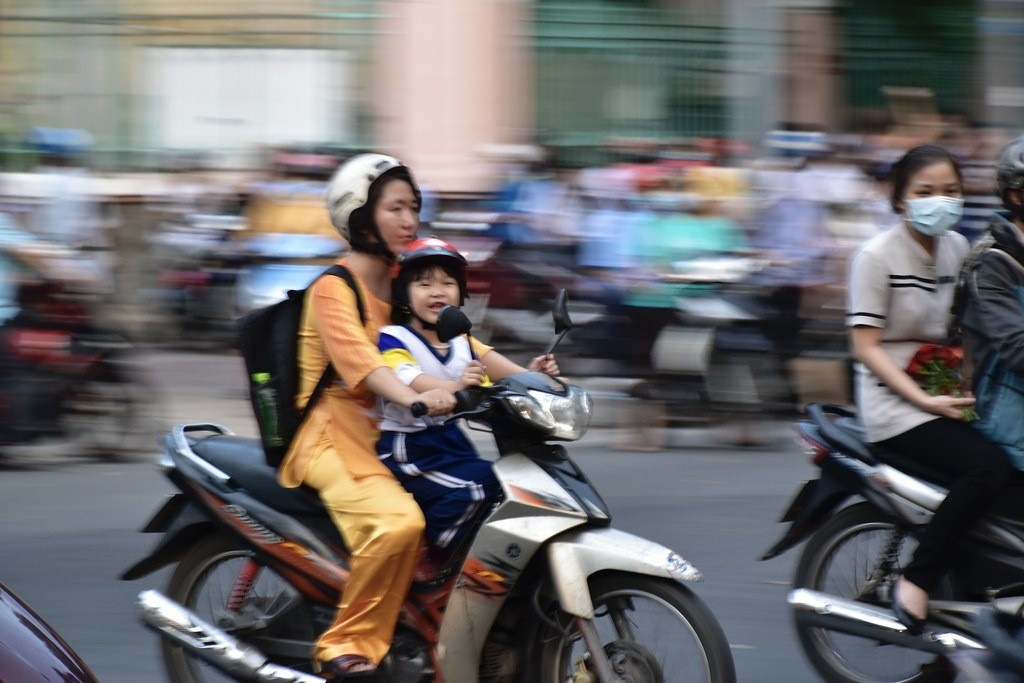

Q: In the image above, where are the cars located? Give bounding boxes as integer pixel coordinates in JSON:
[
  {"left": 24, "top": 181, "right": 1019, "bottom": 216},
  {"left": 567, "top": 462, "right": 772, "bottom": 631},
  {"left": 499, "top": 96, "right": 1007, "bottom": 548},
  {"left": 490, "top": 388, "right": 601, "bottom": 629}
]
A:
[{"left": 1, "top": 2, "right": 1010, "bottom": 443}]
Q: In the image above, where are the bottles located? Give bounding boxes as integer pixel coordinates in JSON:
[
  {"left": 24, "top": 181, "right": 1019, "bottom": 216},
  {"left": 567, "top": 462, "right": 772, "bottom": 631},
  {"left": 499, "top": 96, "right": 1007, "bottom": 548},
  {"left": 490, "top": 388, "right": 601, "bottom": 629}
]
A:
[{"left": 251, "top": 372, "right": 286, "bottom": 447}]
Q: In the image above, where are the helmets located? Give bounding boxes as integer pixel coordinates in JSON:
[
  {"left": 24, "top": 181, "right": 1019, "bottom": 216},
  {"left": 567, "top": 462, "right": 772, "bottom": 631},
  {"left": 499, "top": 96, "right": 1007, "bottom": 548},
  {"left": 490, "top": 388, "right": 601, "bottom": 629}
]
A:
[
  {"left": 391, "top": 237, "right": 468, "bottom": 305},
  {"left": 997, "top": 139, "right": 1024, "bottom": 201},
  {"left": 327, "top": 152, "right": 406, "bottom": 246}
]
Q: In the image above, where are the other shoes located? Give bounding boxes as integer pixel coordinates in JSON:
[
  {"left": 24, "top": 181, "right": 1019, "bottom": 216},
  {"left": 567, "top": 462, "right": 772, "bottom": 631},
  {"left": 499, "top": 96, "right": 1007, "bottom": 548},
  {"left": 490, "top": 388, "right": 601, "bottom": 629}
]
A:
[
  {"left": 891, "top": 575, "right": 929, "bottom": 631},
  {"left": 411, "top": 540, "right": 438, "bottom": 584}
]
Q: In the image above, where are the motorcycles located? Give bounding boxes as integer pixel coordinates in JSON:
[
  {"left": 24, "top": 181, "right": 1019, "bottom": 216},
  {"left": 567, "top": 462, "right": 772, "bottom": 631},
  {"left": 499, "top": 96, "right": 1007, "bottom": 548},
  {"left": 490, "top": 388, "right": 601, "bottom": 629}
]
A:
[
  {"left": 755, "top": 399, "right": 1023, "bottom": 683},
  {"left": 116, "top": 286, "right": 741, "bottom": 682}
]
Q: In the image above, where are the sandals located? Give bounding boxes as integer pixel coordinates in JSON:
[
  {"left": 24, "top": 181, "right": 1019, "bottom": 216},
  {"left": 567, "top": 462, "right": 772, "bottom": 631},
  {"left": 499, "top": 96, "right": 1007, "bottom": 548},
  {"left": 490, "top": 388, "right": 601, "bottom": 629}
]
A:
[{"left": 311, "top": 653, "right": 377, "bottom": 677}]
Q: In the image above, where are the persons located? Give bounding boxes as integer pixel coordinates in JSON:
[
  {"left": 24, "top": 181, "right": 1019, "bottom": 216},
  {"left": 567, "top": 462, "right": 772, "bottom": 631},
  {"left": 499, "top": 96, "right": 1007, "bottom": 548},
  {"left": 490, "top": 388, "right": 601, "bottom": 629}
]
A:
[
  {"left": 0, "top": 77, "right": 1010, "bottom": 475},
  {"left": 277, "top": 154, "right": 568, "bottom": 676},
  {"left": 845, "top": 144, "right": 1015, "bottom": 632},
  {"left": 374, "top": 237, "right": 560, "bottom": 585},
  {"left": 958, "top": 136, "right": 1024, "bottom": 471}
]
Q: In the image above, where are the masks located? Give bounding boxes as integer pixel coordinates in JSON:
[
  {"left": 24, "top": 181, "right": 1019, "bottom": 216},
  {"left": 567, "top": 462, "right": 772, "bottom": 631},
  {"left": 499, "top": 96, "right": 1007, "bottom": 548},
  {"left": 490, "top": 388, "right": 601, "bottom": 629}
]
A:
[{"left": 902, "top": 195, "right": 966, "bottom": 237}]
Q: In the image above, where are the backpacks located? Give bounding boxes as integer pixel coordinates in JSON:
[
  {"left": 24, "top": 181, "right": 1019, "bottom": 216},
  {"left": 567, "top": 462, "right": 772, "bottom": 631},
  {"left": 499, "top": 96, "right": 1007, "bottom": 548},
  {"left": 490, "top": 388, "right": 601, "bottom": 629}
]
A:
[{"left": 236, "top": 264, "right": 364, "bottom": 468}]
